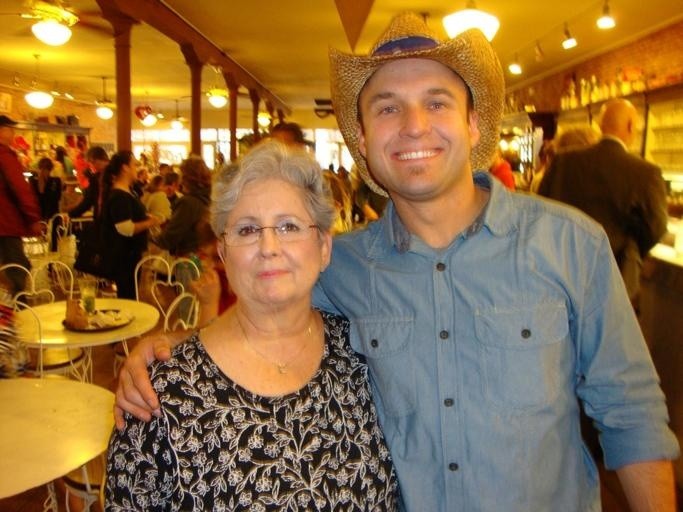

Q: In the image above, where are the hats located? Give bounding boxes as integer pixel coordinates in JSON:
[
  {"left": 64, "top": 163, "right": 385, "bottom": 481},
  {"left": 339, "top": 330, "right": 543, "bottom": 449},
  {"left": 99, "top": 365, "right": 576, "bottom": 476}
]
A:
[{"left": 325, "top": 12, "right": 505, "bottom": 200}]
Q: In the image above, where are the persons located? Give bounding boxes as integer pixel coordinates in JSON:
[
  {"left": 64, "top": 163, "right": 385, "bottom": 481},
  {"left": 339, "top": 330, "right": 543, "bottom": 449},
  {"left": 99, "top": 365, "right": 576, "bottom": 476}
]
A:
[
  {"left": 62, "top": 137, "right": 212, "bottom": 294},
  {"left": 481, "top": 126, "right": 595, "bottom": 195},
  {"left": 0, "top": 115, "right": 42, "bottom": 311},
  {"left": 536, "top": 98, "right": 669, "bottom": 315},
  {"left": 101, "top": 136, "right": 409, "bottom": 512},
  {"left": 29, "top": 143, "right": 69, "bottom": 219},
  {"left": 113, "top": 11, "right": 680, "bottom": 512}
]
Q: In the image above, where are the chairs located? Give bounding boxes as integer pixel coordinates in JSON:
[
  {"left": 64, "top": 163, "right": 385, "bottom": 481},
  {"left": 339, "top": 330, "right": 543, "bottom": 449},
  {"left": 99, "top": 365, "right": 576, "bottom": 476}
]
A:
[
  {"left": 12, "top": 299, "right": 45, "bottom": 378},
  {"left": 1, "top": 260, "right": 93, "bottom": 383},
  {"left": 163, "top": 292, "right": 199, "bottom": 332},
  {"left": 63, "top": 452, "right": 106, "bottom": 512},
  {"left": 113, "top": 254, "right": 201, "bottom": 377},
  {"left": 48, "top": 213, "right": 118, "bottom": 301}
]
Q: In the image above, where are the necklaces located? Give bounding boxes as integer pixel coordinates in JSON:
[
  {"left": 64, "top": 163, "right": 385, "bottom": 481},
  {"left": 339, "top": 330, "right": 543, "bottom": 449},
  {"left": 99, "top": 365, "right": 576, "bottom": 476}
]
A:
[{"left": 232, "top": 304, "right": 314, "bottom": 375}]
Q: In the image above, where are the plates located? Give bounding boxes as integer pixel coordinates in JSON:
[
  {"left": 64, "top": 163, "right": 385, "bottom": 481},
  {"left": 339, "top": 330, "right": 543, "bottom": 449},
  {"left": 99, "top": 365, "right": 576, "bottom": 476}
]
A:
[{"left": 61, "top": 309, "right": 134, "bottom": 334}]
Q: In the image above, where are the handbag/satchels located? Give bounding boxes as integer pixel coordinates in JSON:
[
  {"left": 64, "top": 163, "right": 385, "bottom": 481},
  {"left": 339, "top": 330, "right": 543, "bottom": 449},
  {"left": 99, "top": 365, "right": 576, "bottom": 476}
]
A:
[{"left": 70, "top": 188, "right": 125, "bottom": 286}]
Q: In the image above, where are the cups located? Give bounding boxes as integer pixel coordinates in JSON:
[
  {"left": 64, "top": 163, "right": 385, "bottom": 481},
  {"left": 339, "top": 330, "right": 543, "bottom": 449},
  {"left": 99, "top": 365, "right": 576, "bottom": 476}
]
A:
[{"left": 78, "top": 278, "right": 97, "bottom": 313}]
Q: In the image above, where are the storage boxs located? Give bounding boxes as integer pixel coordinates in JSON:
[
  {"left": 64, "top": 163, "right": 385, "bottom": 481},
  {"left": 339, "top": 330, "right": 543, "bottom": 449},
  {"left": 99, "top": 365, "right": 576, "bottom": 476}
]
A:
[{"left": 23, "top": 53, "right": 54, "bottom": 109}]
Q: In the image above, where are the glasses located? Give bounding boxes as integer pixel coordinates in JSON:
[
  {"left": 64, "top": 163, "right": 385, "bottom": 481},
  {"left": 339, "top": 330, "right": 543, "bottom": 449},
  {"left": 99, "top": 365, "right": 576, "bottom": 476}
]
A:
[{"left": 220, "top": 217, "right": 320, "bottom": 248}]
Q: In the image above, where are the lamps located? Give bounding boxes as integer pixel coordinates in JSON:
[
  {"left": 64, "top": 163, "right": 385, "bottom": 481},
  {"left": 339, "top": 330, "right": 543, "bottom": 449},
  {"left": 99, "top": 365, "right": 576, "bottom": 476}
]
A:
[
  {"left": 24, "top": 0, "right": 80, "bottom": 47},
  {"left": 136, "top": 95, "right": 158, "bottom": 128},
  {"left": 561, "top": 23, "right": 578, "bottom": 50},
  {"left": 441, "top": 0, "right": 502, "bottom": 43},
  {"left": 95, "top": 77, "right": 114, "bottom": 121},
  {"left": 257, "top": 105, "right": 274, "bottom": 128},
  {"left": 170, "top": 99, "right": 187, "bottom": 132},
  {"left": 509, "top": 53, "right": 523, "bottom": 75},
  {"left": 596, "top": 2, "right": 616, "bottom": 31},
  {"left": 205, "top": 83, "right": 229, "bottom": 109}
]
